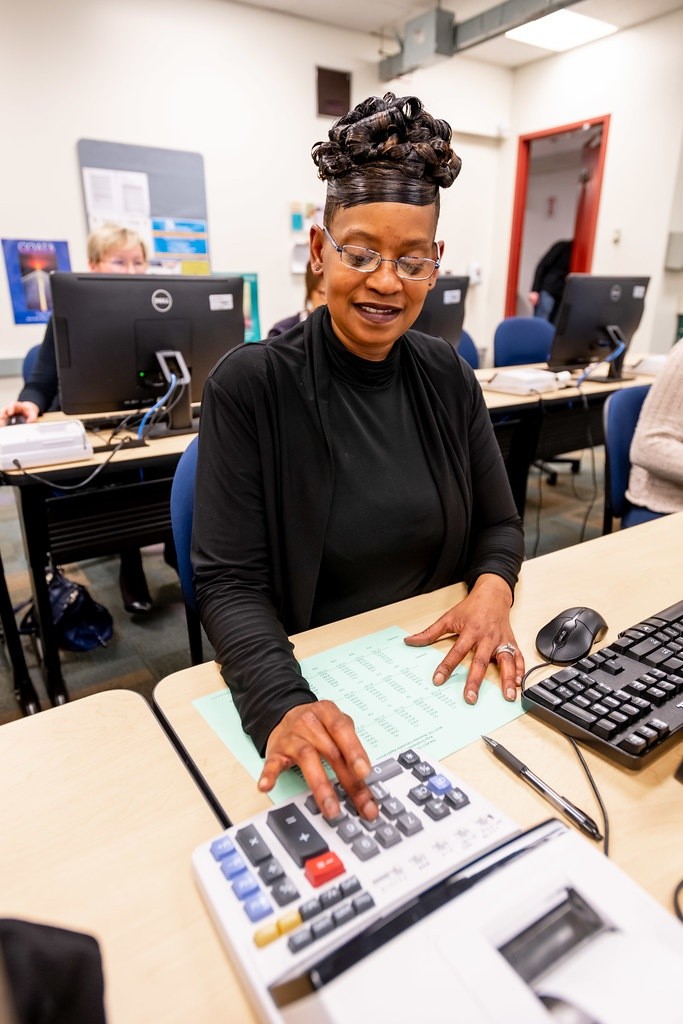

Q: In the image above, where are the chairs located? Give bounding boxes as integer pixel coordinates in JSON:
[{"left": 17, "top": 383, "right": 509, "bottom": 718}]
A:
[
  {"left": 170, "top": 434, "right": 205, "bottom": 664},
  {"left": 457, "top": 331, "right": 479, "bottom": 367},
  {"left": 495, "top": 316, "right": 581, "bottom": 487},
  {"left": 602, "top": 382, "right": 651, "bottom": 534},
  {"left": 23, "top": 345, "right": 62, "bottom": 416}
]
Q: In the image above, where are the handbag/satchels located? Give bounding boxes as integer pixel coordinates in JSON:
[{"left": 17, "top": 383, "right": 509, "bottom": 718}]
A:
[{"left": 21, "top": 567, "right": 114, "bottom": 653}]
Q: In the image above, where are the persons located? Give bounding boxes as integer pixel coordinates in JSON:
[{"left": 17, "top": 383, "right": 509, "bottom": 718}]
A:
[
  {"left": 268, "top": 259, "right": 326, "bottom": 338},
  {"left": 191, "top": 91, "right": 524, "bottom": 821},
  {"left": 624, "top": 337, "right": 683, "bottom": 526},
  {"left": 529, "top": 240, "right": 573, "bottom": 324},
  {"left": 0, "top": 224, "right": 179, "bottom": 614}
]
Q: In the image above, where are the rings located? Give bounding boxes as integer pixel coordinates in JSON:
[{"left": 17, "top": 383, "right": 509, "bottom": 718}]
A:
[{"left": 496, "top": 643, "right": 516, "bottom": 658}]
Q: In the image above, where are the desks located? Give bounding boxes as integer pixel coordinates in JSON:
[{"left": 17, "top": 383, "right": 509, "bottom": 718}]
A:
[
  {"left": 0, "top": 510, "right": 681, "bottom": 1022},
  {"left": 0, "top": 362, "right": 673, "bottom": 717}
]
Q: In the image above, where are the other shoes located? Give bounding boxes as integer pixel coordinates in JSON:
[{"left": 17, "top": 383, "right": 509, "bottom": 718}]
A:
[{"left": 118, "top": 563, "right": 152, "bottom": 615}]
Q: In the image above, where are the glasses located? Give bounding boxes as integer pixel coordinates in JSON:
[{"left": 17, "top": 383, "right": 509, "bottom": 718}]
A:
[
  {"left": 323, "top": 226, "right": 441, "bottom": 280},
  {"left": 96, "top": 257, "right": 145, "bottom": 272}
]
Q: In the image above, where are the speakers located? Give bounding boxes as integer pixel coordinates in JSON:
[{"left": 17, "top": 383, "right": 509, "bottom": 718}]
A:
[{"left": 665, "top": 231, "right": 683, "bottom": 273}]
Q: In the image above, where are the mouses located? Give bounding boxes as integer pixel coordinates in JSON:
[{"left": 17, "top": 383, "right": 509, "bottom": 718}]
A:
[
  {"left": 536, "top": 607, "right": 608, "bottom": 667},
  {"left": 6, "top": 412, "right": 25, "bottom": 425}
]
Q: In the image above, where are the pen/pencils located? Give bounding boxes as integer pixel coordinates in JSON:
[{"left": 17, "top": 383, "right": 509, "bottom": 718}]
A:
[{"left": 481, "top": 734, "right": 604, "bottom": 843}]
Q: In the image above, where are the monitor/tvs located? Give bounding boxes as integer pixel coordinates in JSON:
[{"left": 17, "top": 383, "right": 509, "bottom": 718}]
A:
[
  {"left": 49, "top": 271, "right": 244, "bottom": 441},
  {"left": 548, "top": 273, "right": 651, "bottom": 383},
  {"left": 409, "top": 275, "right": 469, "bottom": 346}
]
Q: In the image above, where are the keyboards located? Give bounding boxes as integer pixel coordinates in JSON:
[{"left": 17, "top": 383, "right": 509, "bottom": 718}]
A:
[
  {"left": 521, "top": 602, "right": 683, "bottom": 771},
  {"left": 545, "top": 364, "right": 588, "bottom": 373},
  {"left": 81, "top": 406, "right": 202, "bottom": 432}
]
